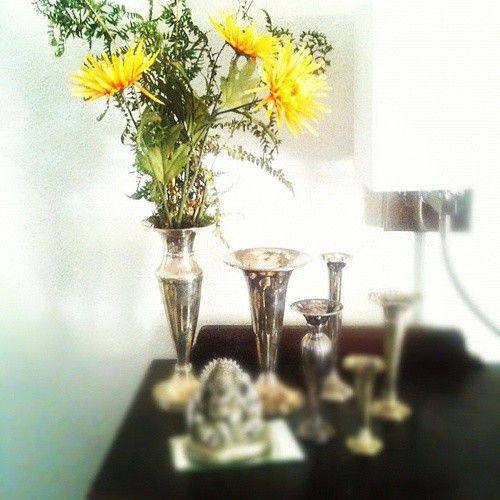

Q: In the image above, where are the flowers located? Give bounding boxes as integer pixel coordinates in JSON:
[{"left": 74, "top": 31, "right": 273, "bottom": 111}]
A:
[{"left": 30, "top": 0, "right": 334, "bottom": 234}]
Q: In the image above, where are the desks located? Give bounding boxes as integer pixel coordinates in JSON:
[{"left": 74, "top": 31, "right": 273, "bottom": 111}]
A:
[{"left": 88, "top": 356, "right": 499, "bottom": 500}]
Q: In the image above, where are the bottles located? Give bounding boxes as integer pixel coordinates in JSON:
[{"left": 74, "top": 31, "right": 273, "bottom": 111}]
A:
[
  {"left": 320, "top": 249, "right": 354, "bottom": 402},
  {"left": 224, "top": 246, "right": 312, "bottom": 417},
  {"left": 141, "top": 217, "right": 219, "bottom": 409},
  {"left": 342, "top": 354, "right": 386, "bottom": 456},
  {"left": 365, "top": 291, "right": 423, "bottom": 421},
  {"left": 290, "top": 298, "right": 344, "bottom": 443}
]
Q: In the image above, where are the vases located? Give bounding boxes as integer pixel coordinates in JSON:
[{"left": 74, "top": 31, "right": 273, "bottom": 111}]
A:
[{"left": 150, "top": 227, "right": 205, "bottom": 410}]
